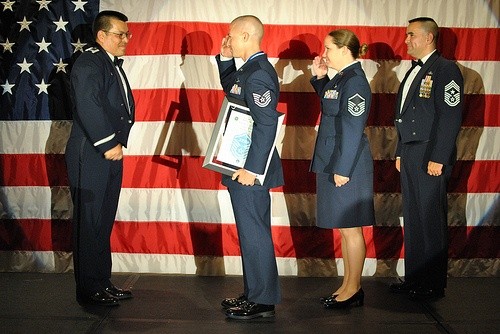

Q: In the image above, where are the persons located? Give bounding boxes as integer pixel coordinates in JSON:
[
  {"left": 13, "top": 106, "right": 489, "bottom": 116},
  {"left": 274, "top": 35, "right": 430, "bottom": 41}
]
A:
[
  {"left": 310, "top": 29, "right": 376, "bottom": 310},
  {"left": 214, "top": 15, "right": 287, "bottom": 320},
  {"left": 65, "top": 10, "right": 136, "bottom": 305},
  {"left": 389, "top": 17, "right": 464, "bottom": 297}
]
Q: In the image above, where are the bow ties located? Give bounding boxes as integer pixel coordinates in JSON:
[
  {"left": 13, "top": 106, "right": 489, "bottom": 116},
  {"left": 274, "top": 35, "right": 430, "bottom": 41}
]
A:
[
  {"left": 412, "top": 59, "right": 423, "bottom": 68},
  {"left": 114, "top": 56, "right": 124, "bottom": 67}
]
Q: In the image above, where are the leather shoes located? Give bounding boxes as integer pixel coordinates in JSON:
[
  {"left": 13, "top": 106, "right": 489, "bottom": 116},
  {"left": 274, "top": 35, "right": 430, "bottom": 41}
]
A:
[
  {"left": 76, "top": 279, "right": 134, "bottom": 307},
  {"left": 221, "top": 296, "right": 276, "bottom": 320}
]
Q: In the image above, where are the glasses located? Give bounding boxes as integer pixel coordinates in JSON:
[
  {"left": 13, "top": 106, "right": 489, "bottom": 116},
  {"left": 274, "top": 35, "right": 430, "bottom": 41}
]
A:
[{"left": 103, "top": 31, "right": 132, "bottom": 39}]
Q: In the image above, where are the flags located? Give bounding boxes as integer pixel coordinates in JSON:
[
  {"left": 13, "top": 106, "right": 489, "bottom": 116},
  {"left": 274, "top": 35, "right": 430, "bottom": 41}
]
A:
[{"left": 0, "top": 0, "right": 500, "bottom": 278}]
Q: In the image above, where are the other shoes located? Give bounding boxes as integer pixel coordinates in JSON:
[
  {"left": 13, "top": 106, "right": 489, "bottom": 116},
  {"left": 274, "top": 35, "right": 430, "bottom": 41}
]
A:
[
  {"left": 410, "top": 288, "right": 445, "bottom": 300},
  {"left": 320, "top": 288, "right": 365, "bottom": 310},
  {"left": 389, "top": 278, "right": 413, "bottom": 295}
]
